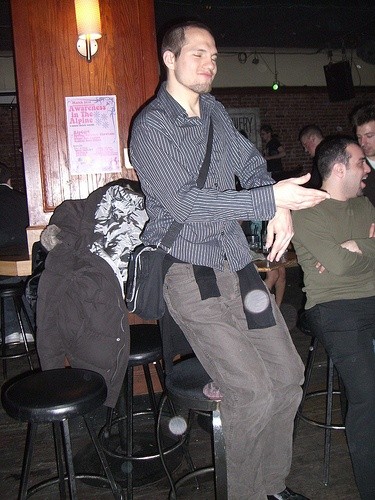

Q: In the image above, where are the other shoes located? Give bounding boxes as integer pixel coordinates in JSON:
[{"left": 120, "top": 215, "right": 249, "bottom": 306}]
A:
[
  {"left": 20, "top": 333, "right": 35, "bottom": 343},
  {"left": 0, "top": 332, "right": 20, "bottom": 346},
  {"left": 267, "top": 486, "right": 310, "bottom": 500}
]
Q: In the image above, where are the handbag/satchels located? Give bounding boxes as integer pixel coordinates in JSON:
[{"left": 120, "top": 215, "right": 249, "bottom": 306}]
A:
[{"left": 126, "top": 244, "right": 174, "bottom": 321}]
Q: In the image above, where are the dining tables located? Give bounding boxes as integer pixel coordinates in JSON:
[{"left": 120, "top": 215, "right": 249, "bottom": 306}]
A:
[{"left": 255, "top": 250, "right": 298, "bottom": 272}]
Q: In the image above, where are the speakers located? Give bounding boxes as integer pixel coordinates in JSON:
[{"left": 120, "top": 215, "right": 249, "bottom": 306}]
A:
[{"left": 324, "top": 61, "right": 355, "bottom": 103}]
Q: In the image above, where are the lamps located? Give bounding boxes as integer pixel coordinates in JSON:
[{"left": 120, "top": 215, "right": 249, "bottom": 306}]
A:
[
  {"left": 74, "top": 0, "right": 103, "bottom": 62},
  {"left": 273, "top": 80, "right": 280, "bottom": 91}
]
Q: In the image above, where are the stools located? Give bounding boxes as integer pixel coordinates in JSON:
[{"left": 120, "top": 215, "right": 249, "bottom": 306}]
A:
[
  {"left": 0, "top": 279, "right": 37, "bottom": 381},
  {"left": 100, "top": 323, "right": 200, "bottom": 500},
  {"left": 155, "top": 356, "right": 227, "bottom": 500},
  {"left": 296, "top": 310, "right": 348, "bottom": 486},
  {"left": 3, "top": 368, "right": 125, "bottom": 500}
]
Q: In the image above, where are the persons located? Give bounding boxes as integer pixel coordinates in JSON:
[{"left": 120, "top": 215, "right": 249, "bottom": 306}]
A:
[
  {"left": 0, "top": 162, "right": 34, "bottom": 345},
  {"left": 234, "top": 105, "right": 375, "bottom": 311},
  {"left": 294, "top": 135, "right": 375, "bottom": 500},
  {"left": 128, "top": 21, "right": 330, "bottom": 500}
]
